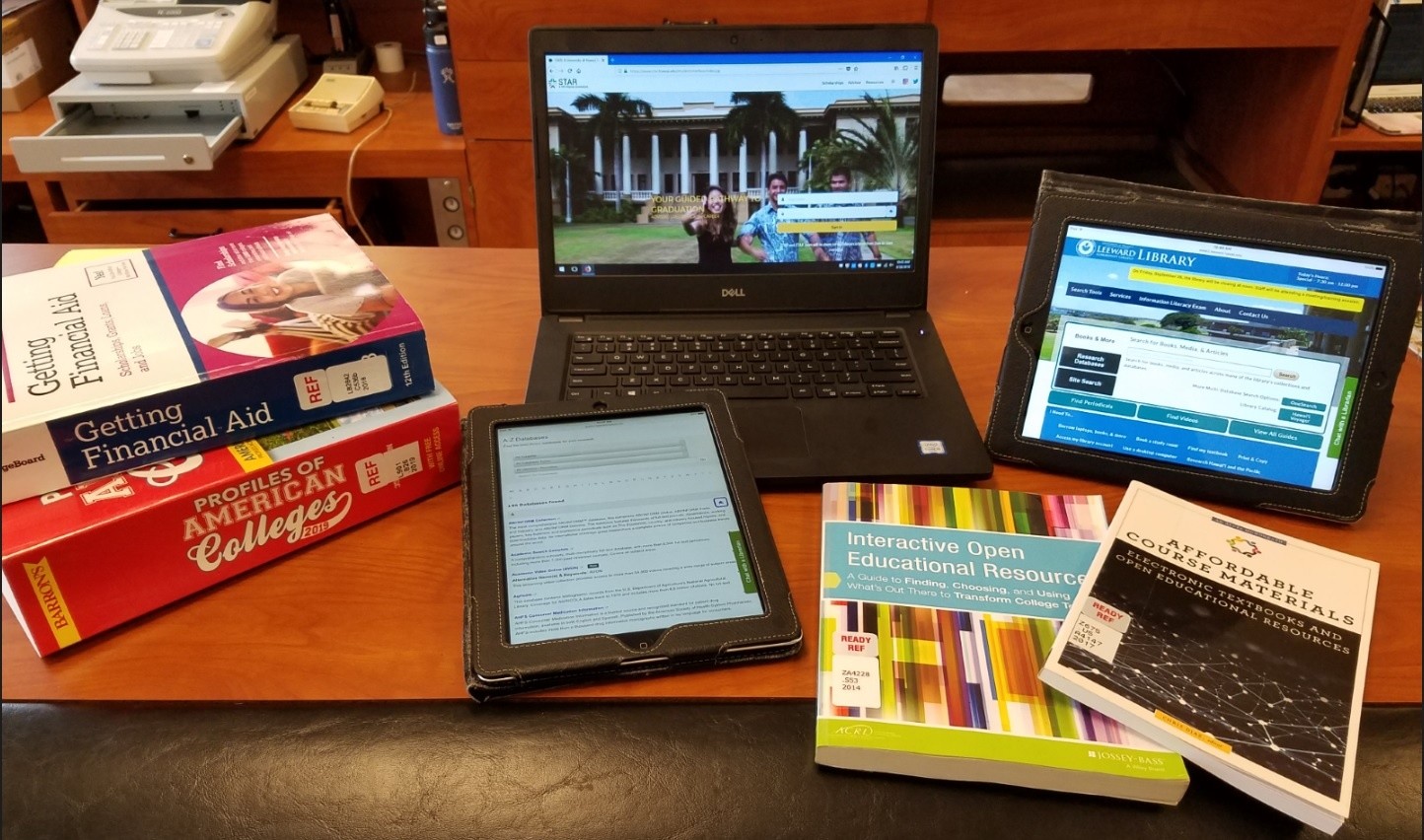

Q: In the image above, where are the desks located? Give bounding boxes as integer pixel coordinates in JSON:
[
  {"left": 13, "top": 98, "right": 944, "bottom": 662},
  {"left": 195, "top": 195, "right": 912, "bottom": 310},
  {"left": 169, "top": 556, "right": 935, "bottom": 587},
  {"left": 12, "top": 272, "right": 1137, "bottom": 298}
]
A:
[{"left": 0, "top": 242, "right": 1424, "bottom": 710}]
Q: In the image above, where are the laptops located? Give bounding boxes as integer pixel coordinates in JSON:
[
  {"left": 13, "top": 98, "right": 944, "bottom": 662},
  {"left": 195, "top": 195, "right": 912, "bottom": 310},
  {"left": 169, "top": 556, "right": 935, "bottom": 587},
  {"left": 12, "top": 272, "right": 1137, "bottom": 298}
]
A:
[
  {"left": 524, "top": 21, "right": 993, "bottom": 488},
  {"left": 1348, "top": 0, "right": 1422, "bottom": 135}
]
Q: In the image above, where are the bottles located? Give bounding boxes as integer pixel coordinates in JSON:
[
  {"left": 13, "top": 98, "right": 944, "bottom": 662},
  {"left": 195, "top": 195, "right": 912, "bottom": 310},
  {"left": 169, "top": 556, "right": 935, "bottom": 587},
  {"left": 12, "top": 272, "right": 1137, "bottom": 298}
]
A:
[
  {"left": 423, "top": 0, "right": 462, "bottom": 135},
  {"left": 1341, "top": 0, "right": 1391, "bottom": 129}
]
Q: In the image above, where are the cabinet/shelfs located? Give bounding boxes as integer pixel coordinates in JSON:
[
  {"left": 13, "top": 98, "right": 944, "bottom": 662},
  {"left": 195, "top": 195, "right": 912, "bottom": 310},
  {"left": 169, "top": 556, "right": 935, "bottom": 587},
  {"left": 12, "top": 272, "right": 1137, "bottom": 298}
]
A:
[{"left": 1, "top": 0, "right": 1424, "bottom": 243}]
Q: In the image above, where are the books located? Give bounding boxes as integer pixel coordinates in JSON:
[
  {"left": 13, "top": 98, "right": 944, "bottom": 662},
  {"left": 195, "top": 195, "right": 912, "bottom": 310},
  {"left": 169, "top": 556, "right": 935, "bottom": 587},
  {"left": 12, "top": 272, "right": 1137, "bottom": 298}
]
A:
[
  {"left": 815, "top": 477, "right": 1381, "bottom": 838},
  {"left": 2, "top": 213, "right": 466, "bottom": 659}
]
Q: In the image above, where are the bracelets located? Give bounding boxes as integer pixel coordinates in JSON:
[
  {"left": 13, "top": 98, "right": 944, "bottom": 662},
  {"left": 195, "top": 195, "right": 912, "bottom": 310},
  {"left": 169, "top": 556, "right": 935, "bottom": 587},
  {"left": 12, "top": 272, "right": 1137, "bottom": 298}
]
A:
[{"left": 874, "top": 254, "right": 881, "bottom": 258}]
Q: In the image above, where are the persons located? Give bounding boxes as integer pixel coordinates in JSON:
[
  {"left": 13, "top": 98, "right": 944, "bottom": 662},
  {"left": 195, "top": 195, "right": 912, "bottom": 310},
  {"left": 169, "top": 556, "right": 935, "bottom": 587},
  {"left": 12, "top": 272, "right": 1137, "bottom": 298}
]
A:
[
  {"left": 682, "top": 167, "right": 884, "bottom": 262},
  {"left": 217, "top": 250, "right": 396, "bottom": 316}
]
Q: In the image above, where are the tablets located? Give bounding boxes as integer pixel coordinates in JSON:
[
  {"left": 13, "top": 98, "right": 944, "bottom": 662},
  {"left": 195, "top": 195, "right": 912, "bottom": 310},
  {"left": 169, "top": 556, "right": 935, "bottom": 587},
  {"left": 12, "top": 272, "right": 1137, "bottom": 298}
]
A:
[
  {"left": 466, "top": 389, "right": 803, "bottom": 683},
  {"left": 986, "top": 187, "right": 1424, "bottom": 527}
]
